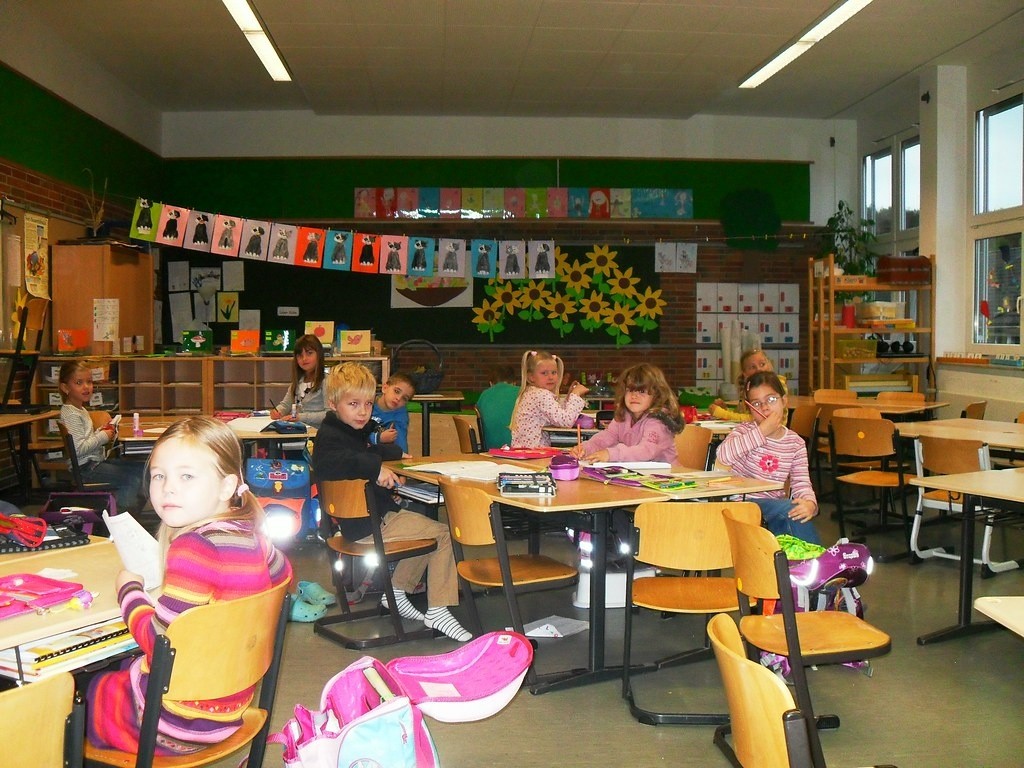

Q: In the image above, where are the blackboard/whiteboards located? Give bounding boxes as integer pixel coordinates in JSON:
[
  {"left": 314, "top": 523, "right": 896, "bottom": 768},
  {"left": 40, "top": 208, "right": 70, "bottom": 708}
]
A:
[{"left": 162, "top": 236, "right": 661, "bottom": 348}]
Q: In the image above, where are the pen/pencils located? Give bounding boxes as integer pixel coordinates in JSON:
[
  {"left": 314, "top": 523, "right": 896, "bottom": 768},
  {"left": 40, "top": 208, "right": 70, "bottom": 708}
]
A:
[
  {"left": 577, "top": 424, "right": 580, "bottom": 460},
  {"left": 745, "top": 399, "right": 767, "bottom": 419},
  {"left": 269, "top": 398, "right": 278, "bottom": 412}
]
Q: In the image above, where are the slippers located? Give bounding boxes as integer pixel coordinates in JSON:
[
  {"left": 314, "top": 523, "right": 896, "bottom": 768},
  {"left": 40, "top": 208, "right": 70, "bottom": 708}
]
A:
[
  {"left": 295, "top": 580, "right": 336, "bottom": 605},
  {"left": 287, "top": 594, "right": 327, "bottom": 622}
]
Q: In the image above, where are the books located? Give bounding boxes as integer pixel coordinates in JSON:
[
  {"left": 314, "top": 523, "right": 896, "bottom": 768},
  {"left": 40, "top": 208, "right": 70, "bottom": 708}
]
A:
[
  {"left": 225, "top": 417, "right": 279, "bottom": 432},
  {"left": 402, "top": 460, "right": 535, "bottom": 482},
  {"left": 695, "top": 420, "right": 739, "bottom": 429},
  {"left": 577, "top": 459, "right": 672, "bottom": 468}
]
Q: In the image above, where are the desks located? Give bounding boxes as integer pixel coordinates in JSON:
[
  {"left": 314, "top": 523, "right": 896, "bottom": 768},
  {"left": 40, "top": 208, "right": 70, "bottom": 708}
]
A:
[
  {"left": 116, "top": 413, "right": 290, "bottom": 422},
  {"left": 789, "top": 394, "right": 950, "bottom": 500},
  {"left": 479, "top": 451, "right": 782, "bottom": 669},
  {"left": 726, "top": 401, "right": 920, "bottom": 504},
  {"left": 408, "top": 391, "right": 464, "bottom": 457},
  {"left": 893, "top": 422, "right": 1024, "bottom": 528},
  {"left": 0, "top": 409, "right": 61, "bottom": 428},
  {"left": 928, "top": 417, "right": 1024, "bottom": 430},
  {"left": 909, "top": 472, "right": 1024, "bottom": 643},
  {"left": 382, "top": 453, "right": 666, "bottom": 697},
  {"left": 116, "top": 423, "right": 321, "bottom": 459},
  {"left": 1001, "top": 466, "right": 1024, "bottom": 473}
]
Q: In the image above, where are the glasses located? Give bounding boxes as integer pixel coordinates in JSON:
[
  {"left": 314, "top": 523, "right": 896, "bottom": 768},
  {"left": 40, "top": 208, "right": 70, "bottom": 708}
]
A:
[{"left": 749, "top": 396, "right": 782, "bottom": 411}]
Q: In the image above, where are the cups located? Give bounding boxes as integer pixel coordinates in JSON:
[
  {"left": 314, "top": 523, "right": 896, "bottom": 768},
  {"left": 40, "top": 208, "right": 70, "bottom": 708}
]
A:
[{"left": 720, "top": 320, "right": 762, "bottom": 383}]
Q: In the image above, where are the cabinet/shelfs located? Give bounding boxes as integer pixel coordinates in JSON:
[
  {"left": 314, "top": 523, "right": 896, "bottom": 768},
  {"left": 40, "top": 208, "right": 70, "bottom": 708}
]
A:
[
  {"left": 809, "top": 256, "right": 938, "bottom": 391},
  {"left": 32, "top": 355, "right": 387, "bottom": 487}
]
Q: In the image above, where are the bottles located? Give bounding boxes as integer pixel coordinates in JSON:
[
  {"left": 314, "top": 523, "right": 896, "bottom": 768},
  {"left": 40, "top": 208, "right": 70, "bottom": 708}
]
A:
[{"left": 842, "top": 299, "right": 855, "bottom": 328}]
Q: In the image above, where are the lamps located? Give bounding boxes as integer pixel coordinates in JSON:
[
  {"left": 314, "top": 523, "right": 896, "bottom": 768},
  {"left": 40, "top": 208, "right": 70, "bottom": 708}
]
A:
[
  {"left": 737, "top": 0, "right": 874, "bottom": 88},
  {"left": 222, "top": 0, "right": 296, "bottom": 82}
]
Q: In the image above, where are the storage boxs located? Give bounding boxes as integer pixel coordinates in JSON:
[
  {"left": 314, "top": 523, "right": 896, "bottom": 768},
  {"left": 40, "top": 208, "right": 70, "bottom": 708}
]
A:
[
  {"left": 827, "top": 272, "right": 867, "bottom": 284},
  {"left": 855, "top": 300, "right": 906, "bottom": 328},
  {"left": 836, "top": 339, "right": 877, "bottom": 358}
]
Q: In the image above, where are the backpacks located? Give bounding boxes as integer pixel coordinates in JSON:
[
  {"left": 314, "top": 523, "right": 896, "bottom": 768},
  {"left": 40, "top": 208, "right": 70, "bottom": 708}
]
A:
[
  {"left": 239, "top": 632, "right": 532, "bottom": 768},
  {"left": 760, "top": 535, "right": 873, "bottom": 684},
  {"left": 40, "top": 492, "right": 115, "bottom": 538},
  {"left": 243, "top": 422, "right": 321, "bottom": 543}
]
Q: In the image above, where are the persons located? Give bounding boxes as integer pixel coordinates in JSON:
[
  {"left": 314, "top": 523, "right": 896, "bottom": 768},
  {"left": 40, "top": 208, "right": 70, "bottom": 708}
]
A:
[
  {"left": 570, "top": 363, "right": 684, "bottom": 464},
  {"left": 709, "top": 350, "right": 788, "bottom": 427},
  {"left": 369, "top": 372, "right": 415, "bottom": 484},
  {"left": 271, "top": 335, "right": 331, "bottom": 460},
  {"left": 715, "top": 371, "right": 819, "bottom": 546},
  {"left": 59, "top": 360, "right": 149, "bottom": 516},
  {"left": 83, "top": 414, "right": 288, "bottom": 768},
  {"left": 476, "top": 365, "right": 520, "bottom": 450},
  {"left": 314, "top": 362, "right": 472, "bottom": 641},
  {"left": 508, "top": 350, "right": 589, "bottom": 448}
]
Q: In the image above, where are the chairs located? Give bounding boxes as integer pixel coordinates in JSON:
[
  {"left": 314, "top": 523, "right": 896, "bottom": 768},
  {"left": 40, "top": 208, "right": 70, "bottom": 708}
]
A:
[
  {"left": 0, "top": 392, "right": 1024, "bottom": 768},
  {"left": 0, "top": 297, "right": 52, "bottom": 414}
]
